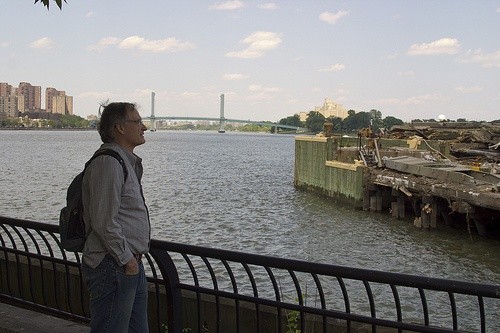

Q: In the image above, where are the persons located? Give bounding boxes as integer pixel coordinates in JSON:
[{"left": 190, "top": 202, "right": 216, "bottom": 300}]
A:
[{"left": 81, "top": 97, "right": 152, "bottom": 333}]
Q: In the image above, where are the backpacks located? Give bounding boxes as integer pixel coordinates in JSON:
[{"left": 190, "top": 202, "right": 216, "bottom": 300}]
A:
[{"left": 60, "top": 148, "right": 128, "bottom": 252}]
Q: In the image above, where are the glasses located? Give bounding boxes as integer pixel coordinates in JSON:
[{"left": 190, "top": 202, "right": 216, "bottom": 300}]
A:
[{"left": 126, "top": 118, "right": 144, "bottom": 125}]
